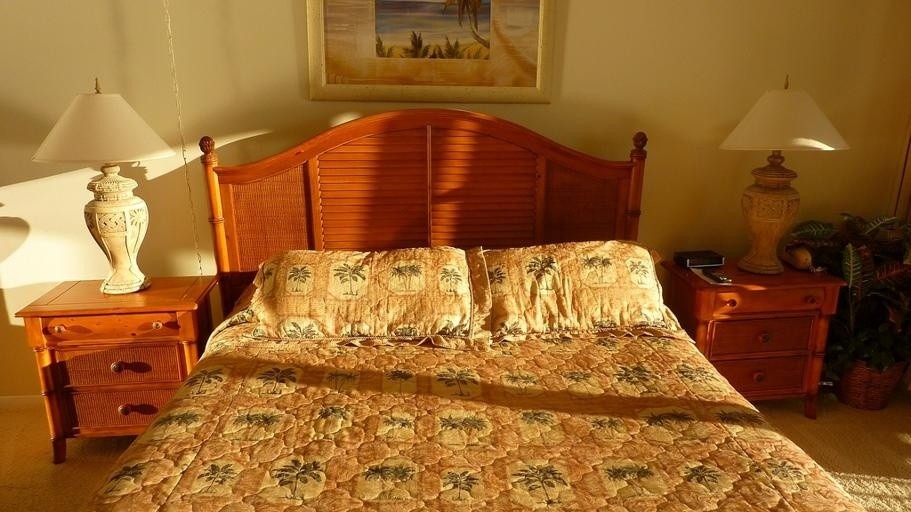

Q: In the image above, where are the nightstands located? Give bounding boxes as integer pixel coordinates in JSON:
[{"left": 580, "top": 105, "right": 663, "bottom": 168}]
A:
[
  {"left": 659, "top": 258, "right": 848, "bottom": 421},
  {"left": 13, "top": 276, "right": 221, "bottom": 464}
]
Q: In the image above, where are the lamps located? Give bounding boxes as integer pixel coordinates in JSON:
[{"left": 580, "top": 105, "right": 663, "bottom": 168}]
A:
[
  {"left": 720, "top": 73, "right": 852, "bottom": 275},
  {"left": 33, "top": 78, "right": 178, "bottom": 295}
]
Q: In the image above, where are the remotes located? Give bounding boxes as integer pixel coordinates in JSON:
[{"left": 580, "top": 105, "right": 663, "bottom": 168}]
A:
[{"left": 703, "top": 268, "right": 733, "bottom": 283}]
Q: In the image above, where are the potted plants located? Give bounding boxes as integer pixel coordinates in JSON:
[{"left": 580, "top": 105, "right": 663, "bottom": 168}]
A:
[{"left": 789, "top": 209, "right": 911, "bottom": 410}]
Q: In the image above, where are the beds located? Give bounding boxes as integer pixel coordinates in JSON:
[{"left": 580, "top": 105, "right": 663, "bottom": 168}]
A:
[{"left": 79, "top": 108, "right": 866, "bottom": 512}]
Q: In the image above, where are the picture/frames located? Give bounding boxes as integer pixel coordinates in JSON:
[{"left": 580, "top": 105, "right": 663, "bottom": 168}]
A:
[{"left": 306, "top": 1, "right": 558, "bottom": 105}]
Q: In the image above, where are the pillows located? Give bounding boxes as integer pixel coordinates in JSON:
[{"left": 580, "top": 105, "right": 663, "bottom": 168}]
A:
[
  {"left": 482, "top": 239, "right": 697, "bottom": 346},
  {"left": 249, "top": 245, "right": 493, "bottom": 353}
]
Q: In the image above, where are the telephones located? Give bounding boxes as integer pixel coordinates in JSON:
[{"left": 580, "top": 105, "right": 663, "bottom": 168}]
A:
[{"left": 779, "top": 242, "right": 811, "bottom": 269}]
